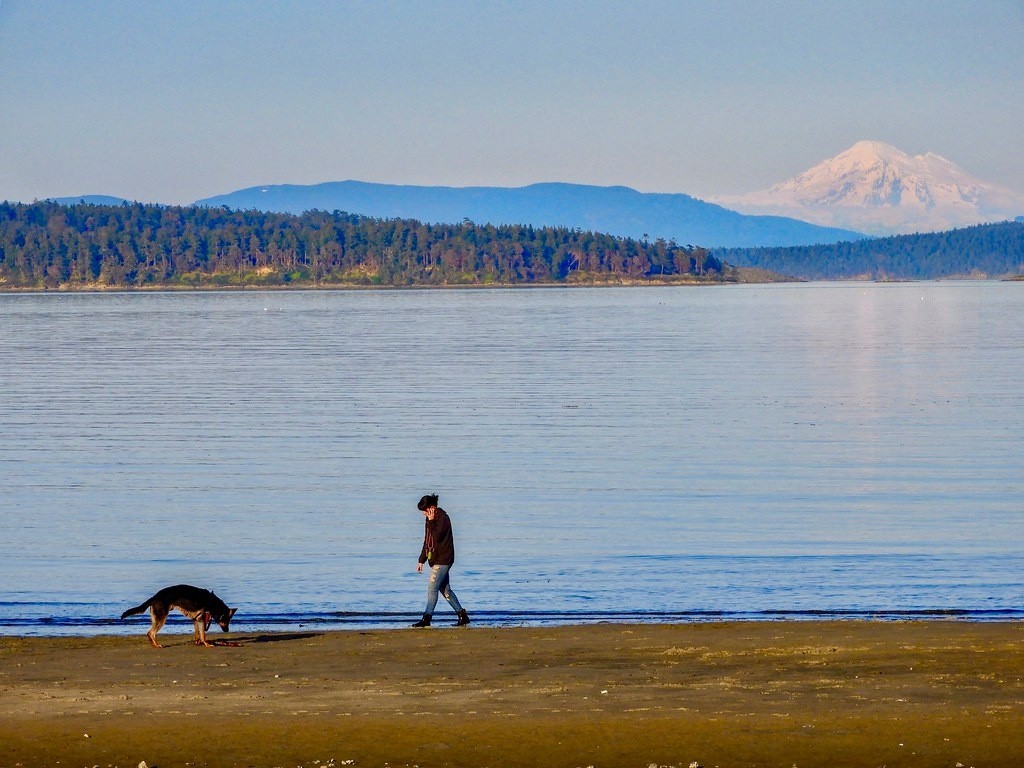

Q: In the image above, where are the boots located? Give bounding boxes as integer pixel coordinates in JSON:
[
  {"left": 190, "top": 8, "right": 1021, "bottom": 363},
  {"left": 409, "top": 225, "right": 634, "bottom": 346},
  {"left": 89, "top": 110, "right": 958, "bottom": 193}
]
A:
[
  {"left": 412, "top": 613, "right": 432, "bottom": 627},
  {"left": 451, "top": 609, "right": 470, "bottom": 627}
]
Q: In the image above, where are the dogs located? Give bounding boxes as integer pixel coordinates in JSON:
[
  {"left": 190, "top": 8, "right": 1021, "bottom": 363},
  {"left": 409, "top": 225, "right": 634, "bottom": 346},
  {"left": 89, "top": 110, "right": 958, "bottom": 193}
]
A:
[{"left": 120, "top": 585, "right": 237, "bottom": 649}]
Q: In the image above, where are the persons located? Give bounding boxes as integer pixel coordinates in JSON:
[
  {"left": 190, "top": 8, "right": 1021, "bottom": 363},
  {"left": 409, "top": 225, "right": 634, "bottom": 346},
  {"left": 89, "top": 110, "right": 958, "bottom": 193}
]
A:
[{"left": 411, "top": 493, "right": 471, "bottom": 627}]
